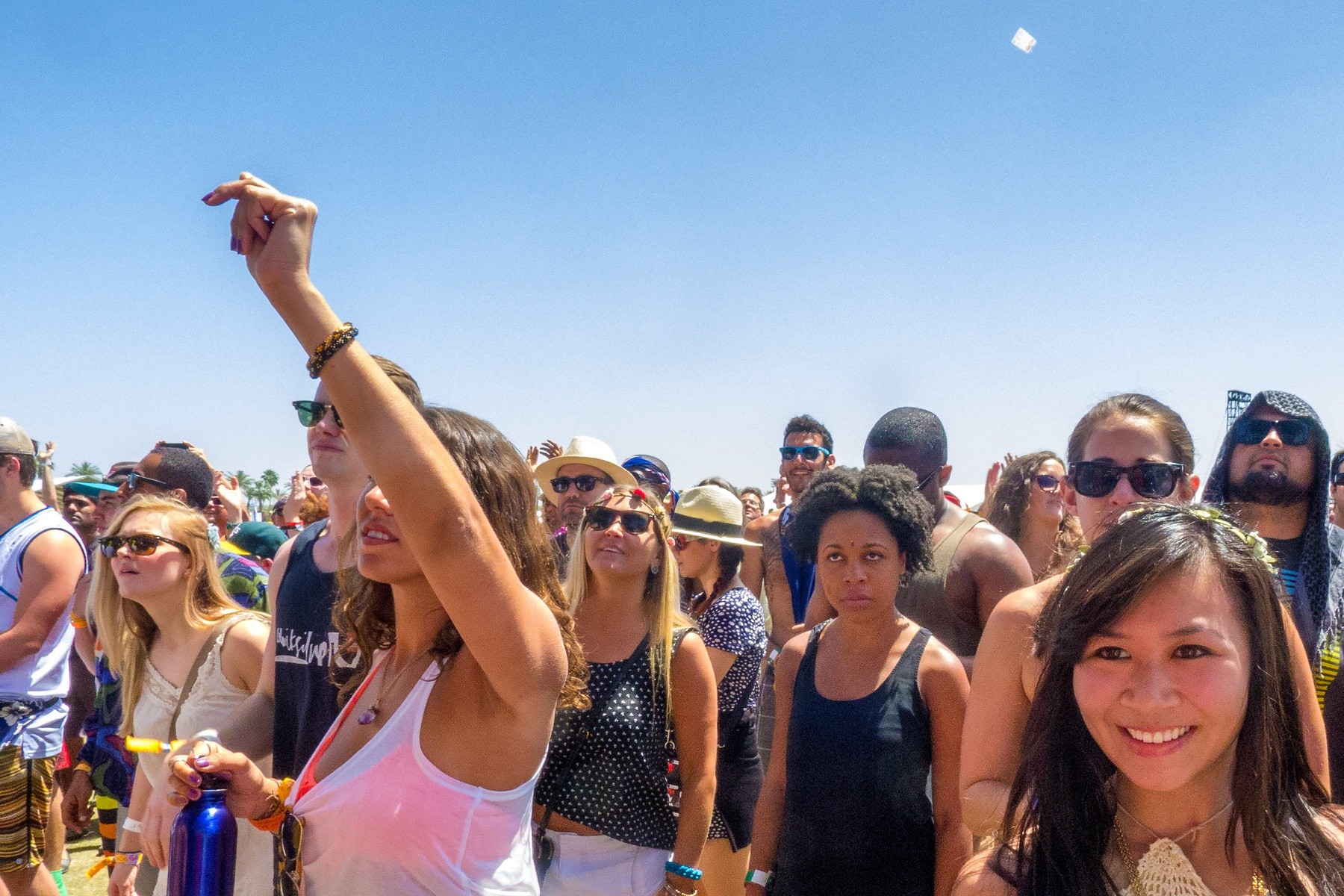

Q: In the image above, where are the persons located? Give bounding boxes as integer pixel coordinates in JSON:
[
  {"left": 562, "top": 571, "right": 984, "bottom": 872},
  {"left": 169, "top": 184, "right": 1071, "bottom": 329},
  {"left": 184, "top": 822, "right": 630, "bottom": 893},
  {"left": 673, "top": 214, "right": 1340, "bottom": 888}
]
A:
[
  {"left": 664, "top": 861, "right": 701, "bottom": 880},
  {"left": 0, "top": 354, "right": 1344, "bottom": 896},
  {"left": 163, "top": 173, "right": 589, "bottom": 896}
]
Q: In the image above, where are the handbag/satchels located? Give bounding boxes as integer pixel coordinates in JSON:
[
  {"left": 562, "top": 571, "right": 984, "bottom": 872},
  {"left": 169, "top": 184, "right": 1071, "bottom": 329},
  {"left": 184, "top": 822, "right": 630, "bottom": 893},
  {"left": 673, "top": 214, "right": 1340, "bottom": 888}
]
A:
[{"left": 533, "top": 833, "right": 554, "bottom": 888}]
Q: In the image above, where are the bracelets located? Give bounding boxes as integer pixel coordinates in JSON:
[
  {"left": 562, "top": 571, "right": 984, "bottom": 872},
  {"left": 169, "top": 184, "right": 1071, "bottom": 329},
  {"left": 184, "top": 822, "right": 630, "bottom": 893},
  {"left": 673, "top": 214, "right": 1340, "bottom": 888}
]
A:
[
  {"left": 306, "top": 322, "right": 353, "bottom": 370},
  {"left": 70, "top": 610, "right": 86, "bottom": 622},
  {"left": 743, "top": 870, "right": 775, "bottom": 889},
  {"left": 286, "top": 527, "right": 295, "bottom": 529},
  {"left": 41, "top": 461, "right": 52, "bottom": 466},
  {"left": 71, "top": 764, "right": 91, "bottom": 776},
  {"left": 70, "top": 621, "right": 89, "bottom": 628},
  {"left": 85, "top": 852, "right": 143, "bottom": 880},
  {"left": 225, "top": 521, "right": 240, "bottom": 529},
  {"left": 286, "top": 519, "right": 303, "bottom": 526},
  {"left": 310, "top": 328, "right": 358, "bottom": 380},
  {"left": 662, "top": 882, "right": 698, "bottom": 896},
  {"left": 248, "top": 778, "right": 294, "bottom": 831},
  {"left": 122, "top": 816, "right": 142, "bottom": 833}
]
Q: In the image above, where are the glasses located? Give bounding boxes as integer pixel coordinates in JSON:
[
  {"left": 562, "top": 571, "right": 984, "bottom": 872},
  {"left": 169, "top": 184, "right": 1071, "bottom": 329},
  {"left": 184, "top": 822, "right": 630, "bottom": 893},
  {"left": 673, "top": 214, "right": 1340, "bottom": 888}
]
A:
[
  {"left": 1067, "top": 459, "right": 1187, "bottom": 499},
  {"left": 125, "top": 473, "right": 177, "bottom": 495},
  {"left": 542, "top": 498, "right": 550, "bottom": 506},
  {"left": 273, "top": 507, "right": 284, "bottom": 517},
  {"left": 302, "top": 476, "right": 326, "bottom": 490},
  {"left": 674, "top": 535, "right": 706, "bottom": 551},
  {"left": 1334, "top": 473, "right": 1344, "bottom": 486},
  {"left": 292, "top": 398, "right": 345, "bottom": 429},
  {"left": 584, "top": 506, "right": 663, "bottom": 547},
  {"left": 778, "top": 445, "right": 831, "bottom": 461},
  {"left": 273, "top": 810, "right": 306, "bottom": 895},
  {"left": 1234, "top": 417, "right": 1312, "bottom": 447},
  {"left": 632, "top": 470, "right": 664, "bottom": 486},
  {"left": 1030, "top": 473, "right": 1067, "bottom": 494},
  {"left": 97, "top": 534, "right": 192, "bottom": 558},
  {"left": 551, "top": 475, "right": 610, "bottom": 492},
  {"left": 208, "top": 497, "right": 223, "bottom": 505}
]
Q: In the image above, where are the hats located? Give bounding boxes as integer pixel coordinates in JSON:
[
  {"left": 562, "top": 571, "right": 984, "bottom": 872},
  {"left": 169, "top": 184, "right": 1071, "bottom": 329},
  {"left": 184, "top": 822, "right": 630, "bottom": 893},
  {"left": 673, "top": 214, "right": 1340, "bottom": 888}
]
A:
[
  {"left": 944, "top": 491, "right": 961, "bottom": 508},
  {"left": 671, "top": 486, "right": 765, "bottom": 546},
  {"left": 0, "top": 416, "right": 36, "bottom": 455},
  {"left": 218, "top": 522, "right": 289, "bottom": 562},
  {"left": 533, "top": 435, "right": 638, "bottom": 506},
  {"left": 70, "top": 461, "right": 138, "bottom": 498}
]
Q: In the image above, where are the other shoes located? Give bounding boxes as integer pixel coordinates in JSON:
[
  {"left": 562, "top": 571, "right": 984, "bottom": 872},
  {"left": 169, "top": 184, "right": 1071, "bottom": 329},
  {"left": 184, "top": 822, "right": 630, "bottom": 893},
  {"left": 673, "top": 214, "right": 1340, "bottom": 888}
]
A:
[
  {"left": 61, "top": 847, "right": 70, "bottom": 872},
  {"left": 65, "top": 826, "right": 90, "bottom": 841}
]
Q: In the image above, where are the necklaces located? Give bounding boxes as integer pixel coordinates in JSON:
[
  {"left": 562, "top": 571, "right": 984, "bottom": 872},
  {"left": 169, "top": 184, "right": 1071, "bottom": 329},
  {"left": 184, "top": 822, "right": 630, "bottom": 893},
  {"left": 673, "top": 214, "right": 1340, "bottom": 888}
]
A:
[
  {"left": 1118, "top": 800, "right": 1233, "bottom": 896},
  {"left": 358, "top": 650, "right": 429, "bottom": 724},
  {"left": 1111, "top": 815, "right": 1267, "bottom": 896}
]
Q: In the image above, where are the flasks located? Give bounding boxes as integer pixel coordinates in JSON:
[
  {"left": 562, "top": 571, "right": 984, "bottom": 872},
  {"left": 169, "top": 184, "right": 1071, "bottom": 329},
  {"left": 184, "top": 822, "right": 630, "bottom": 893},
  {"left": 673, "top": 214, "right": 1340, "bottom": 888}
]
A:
[{"left": 165, "top": 770, "right": 238, "bottom": 896}]
